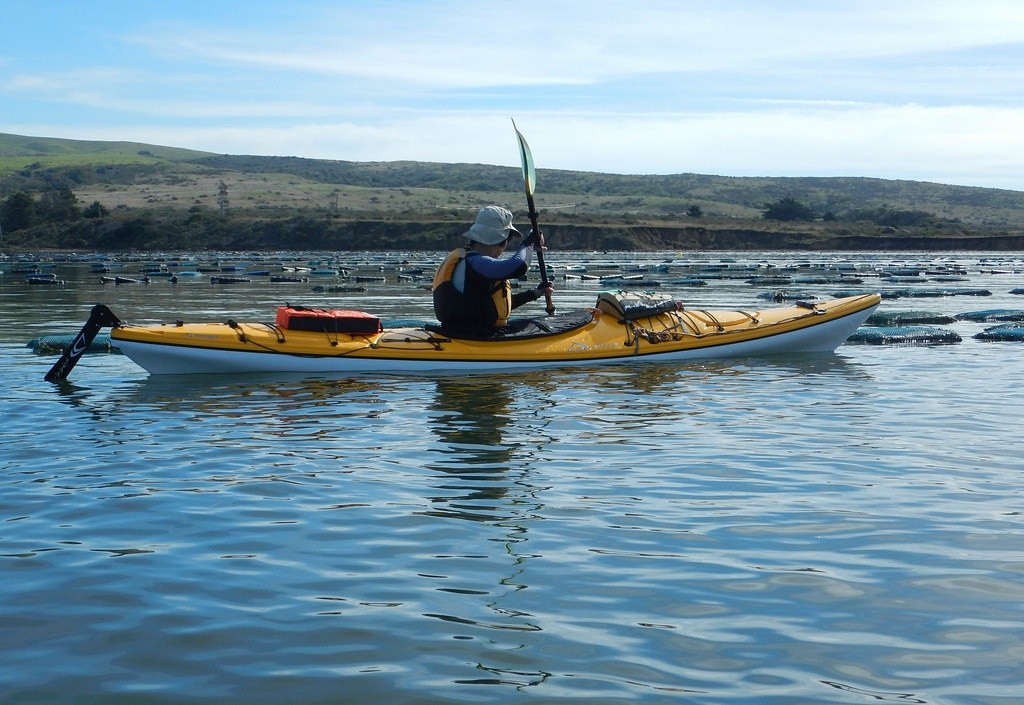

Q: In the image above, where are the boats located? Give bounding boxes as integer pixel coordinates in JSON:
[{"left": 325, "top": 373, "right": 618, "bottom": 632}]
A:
[{"left": 36, "top": 291, "right": 884, "bottom": 387}]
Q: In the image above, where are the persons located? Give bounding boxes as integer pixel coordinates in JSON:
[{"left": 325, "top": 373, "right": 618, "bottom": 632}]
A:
[{"left": 431, "top": 205, "right": 555, "bottom": 344}]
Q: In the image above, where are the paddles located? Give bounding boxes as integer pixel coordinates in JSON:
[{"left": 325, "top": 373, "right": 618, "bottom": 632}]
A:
[{"left": 511, "top": 117, "right": 557, "bottom": 317}]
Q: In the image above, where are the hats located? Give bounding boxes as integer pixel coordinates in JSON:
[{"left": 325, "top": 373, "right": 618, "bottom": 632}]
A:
[{"left": 462, "top": 205, "right": 523, "bottom": 245}]
